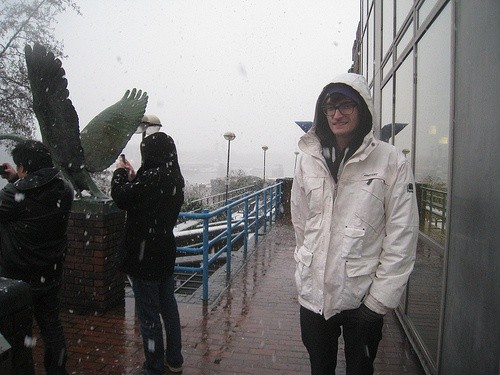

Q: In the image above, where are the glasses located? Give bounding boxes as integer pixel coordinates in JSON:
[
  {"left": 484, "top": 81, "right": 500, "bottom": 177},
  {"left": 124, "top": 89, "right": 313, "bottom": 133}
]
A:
[{"left": 322, "top": 101, "right": 357, "bottom": 116}]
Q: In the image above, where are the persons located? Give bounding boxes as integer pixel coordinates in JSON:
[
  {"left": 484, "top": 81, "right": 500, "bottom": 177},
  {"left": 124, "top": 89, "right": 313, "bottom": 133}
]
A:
[
  {"left": 110, "top": 131, "right": 185, "bottom": 375},
  {"left": 290, "top": 72, "right": 420, "bottom": 375},
  {"left": 0, "top": 139, "right": 74, "bottom": 375}
]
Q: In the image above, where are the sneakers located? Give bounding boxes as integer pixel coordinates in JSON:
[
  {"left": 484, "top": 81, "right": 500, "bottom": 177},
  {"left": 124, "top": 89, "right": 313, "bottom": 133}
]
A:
[{"left": 140, "top": 351, "right": 183, "bottom": 375}]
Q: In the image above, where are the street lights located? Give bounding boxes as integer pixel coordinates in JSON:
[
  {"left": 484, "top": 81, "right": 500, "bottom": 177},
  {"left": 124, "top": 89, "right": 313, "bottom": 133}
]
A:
[
  {"left": 223, "top": 132, "right": 237, "bottom": 204},
  {"left": 294, "top": 151, "right": 300, "bottom": 174},
  {"left": 261, "top": 145, "right": 268, "bottom": 188},
  {"left": 133, "top": 115, "right": 162, "bottom": 142}
]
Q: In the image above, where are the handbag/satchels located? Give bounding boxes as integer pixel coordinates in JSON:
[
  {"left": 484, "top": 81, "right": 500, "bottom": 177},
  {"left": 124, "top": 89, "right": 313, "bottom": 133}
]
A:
[{"left": 120, "top": 238, "right": 160, "bottom": 279}]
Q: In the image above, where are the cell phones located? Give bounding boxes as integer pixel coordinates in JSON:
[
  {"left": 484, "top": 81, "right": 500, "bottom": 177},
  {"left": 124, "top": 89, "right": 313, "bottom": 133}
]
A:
[{"left": 121, "top": 154, "right": 125, "bottom": 164}]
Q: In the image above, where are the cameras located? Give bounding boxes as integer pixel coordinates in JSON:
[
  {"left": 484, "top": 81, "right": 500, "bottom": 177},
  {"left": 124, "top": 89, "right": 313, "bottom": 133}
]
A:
[{"left": 0, "top": 166, "right": 8, "bottom": 178}]
